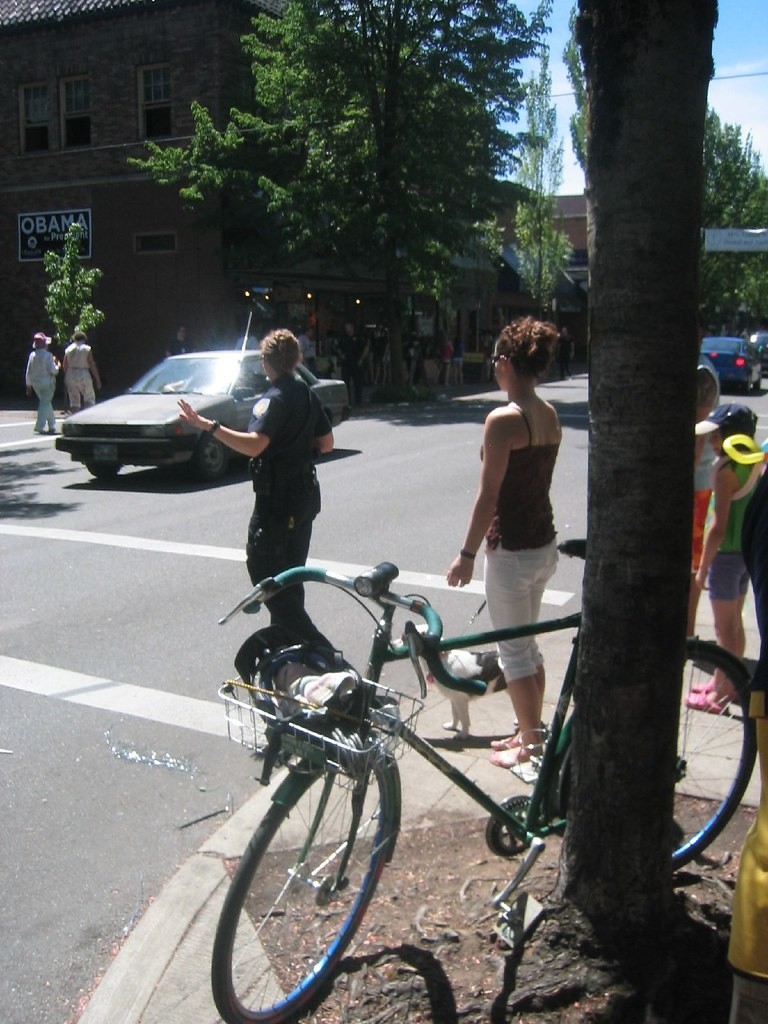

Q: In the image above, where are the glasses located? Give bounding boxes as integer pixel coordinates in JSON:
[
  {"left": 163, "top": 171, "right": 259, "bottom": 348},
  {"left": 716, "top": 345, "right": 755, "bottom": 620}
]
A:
[{"left": 491, "top": 354, "right": 513, "bottom": 367}]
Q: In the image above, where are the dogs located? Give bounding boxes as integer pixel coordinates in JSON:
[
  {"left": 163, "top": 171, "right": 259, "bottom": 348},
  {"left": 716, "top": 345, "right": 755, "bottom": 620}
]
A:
[{"left": 389, "top": 624, "right": 519, "bottom": 740}]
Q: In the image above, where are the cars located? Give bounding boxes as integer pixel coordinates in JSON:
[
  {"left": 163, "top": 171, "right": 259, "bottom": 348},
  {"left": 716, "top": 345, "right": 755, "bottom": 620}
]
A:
[
  {"left": 697, "top": 336, "right": 765, "bottom": 396},
  {"left": 748, "top": 329, "right": 768, "bottom": 374},
  {"left": 55, "top": 348, "right": 353, "bottom": 483}
]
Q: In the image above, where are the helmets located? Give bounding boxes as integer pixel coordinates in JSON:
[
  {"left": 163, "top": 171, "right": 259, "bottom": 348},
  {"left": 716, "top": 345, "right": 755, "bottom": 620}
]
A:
[{"left": 234, "top": 624, "right": 368, "bottom": 731}]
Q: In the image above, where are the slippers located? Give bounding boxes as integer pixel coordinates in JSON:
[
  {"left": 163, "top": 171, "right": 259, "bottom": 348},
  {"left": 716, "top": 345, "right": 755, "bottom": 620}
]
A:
[
  {"left": 692, "top": 683, "right": 735, "bottom": 700},
  {"left": 687, "top": 689, "right": 728, "bottom": 714}
]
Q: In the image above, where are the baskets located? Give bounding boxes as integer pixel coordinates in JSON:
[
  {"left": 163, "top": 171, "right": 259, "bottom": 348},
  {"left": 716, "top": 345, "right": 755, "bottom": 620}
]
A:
[{"left": 215, "top": 674, "right": 424, "bottom": 794}]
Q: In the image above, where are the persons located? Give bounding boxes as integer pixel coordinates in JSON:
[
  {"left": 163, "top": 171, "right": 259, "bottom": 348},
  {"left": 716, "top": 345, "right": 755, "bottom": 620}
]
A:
[
  {"left": 26, "top": 332, "right": 61, "bottom": 435},
  {"left": 689, "top": 314, "right": 768, "bottom": 1023},
  {"left": 177, "top": 329, "right": 334, "bottom": 647},
  {"left": 447, "top": 316, "right": 561, "bottom": 768},
  {"left": 63, "top": 332, "right": 101, "bottom": 415},
  {"left": 165, "top": 320, "right": 575, "bottom": 404}
]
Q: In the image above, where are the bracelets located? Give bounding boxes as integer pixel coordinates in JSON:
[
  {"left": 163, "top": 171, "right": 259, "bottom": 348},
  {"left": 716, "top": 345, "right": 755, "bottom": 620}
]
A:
[
  {"left": 209, "top": 420, "right": 219, "bottom": 434},
  {"left": 459, "top": 550, "right": 475, "bottom": 559}
]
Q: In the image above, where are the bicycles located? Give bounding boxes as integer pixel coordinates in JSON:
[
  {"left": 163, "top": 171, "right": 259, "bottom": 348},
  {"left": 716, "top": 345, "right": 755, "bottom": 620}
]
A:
[{"left": 211, "top": 540, "right": 757, "bottom": 1024}]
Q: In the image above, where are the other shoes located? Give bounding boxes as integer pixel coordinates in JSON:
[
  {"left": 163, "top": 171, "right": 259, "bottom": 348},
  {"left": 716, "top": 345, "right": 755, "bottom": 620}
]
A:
[
  {"left": 49, "top": 429, "right": 55, "bottom": 434},
  {"left": 491, "top": 737, "right": 516, "bottom": 750},
  {"left": 34, "top": 428, "right": 46, "bottom": 434},
  {"left": 489, "top": 747, "right": 523, "bottom": 769}
]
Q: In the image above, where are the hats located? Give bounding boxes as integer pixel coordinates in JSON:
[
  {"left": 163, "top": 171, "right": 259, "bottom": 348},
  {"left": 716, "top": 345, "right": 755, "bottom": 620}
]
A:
[
  {"left": 32, "top": 332, "right": 52, "bottom": 348},
  {"left": 694, "top": 403, "right": 757, "bottom": 435}
]
